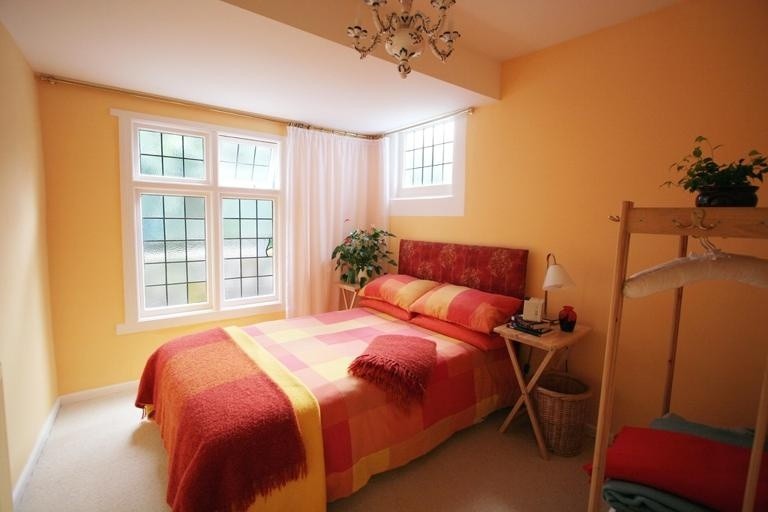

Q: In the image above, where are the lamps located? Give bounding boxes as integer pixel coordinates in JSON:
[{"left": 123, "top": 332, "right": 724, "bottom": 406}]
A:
[
  {"left": 492, "top": 316, "right": 592, "bottom": 460},
  {"left": 347, "top": 0, "right": 460, "bottom": 77},
  {"left": 541, "top": 251, "right": 575, "bottom": 324}
]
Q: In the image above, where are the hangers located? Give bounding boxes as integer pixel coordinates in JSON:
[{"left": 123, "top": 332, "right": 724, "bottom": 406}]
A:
[{"left": 623, "top": 238, "right": 768, "bottom": 302}]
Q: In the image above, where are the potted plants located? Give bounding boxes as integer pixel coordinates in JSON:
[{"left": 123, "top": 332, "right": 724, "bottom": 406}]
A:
[{"left": 659, "top": 132, "right": 768, "bottom": 208}]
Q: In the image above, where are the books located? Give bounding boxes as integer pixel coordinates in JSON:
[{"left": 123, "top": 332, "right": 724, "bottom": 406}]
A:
[
  {"left": 511, "top": 313, "right": 552, "bottom": 330},
  {"left": 510, "top": 320, "right": 550, "bottom": 333},
  {"left": 506, "top": 322, "right": 556, "bottom": 337}
]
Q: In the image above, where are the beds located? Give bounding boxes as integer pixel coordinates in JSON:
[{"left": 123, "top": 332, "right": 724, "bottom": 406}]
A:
[{"left": 135, "top": 240, "right": 531, "bottom": 512}]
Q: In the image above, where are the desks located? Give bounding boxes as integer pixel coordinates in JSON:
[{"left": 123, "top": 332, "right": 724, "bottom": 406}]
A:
[{"left": 337, "top": 283, "right": 361, "bottom": 310}]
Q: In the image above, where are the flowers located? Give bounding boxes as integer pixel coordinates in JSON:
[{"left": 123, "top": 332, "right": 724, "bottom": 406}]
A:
[{"left": 330, "top": 225, "right": 399, "bottom": 288}]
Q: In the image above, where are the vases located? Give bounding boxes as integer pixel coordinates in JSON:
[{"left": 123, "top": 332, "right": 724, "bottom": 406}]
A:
[{"left": 358, "top": 264, "right": 371, "bottom": 286}]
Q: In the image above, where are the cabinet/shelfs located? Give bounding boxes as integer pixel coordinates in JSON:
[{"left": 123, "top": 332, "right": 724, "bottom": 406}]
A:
[{"left": 587, "top": 200, "right": 768, "bottom": 512}]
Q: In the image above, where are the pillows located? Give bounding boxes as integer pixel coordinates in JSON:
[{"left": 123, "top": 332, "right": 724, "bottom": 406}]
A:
[{"left": 360, "top": 273, "right": 523, "bottom": 335}]
